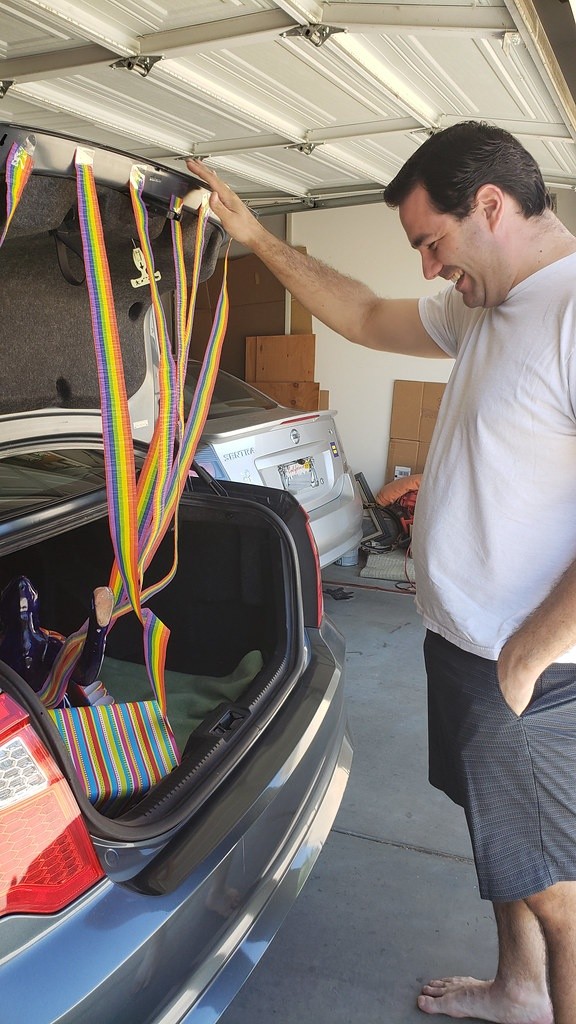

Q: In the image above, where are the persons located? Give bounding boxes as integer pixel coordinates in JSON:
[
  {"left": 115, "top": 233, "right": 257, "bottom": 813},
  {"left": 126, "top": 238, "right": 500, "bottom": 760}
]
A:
[{"left": 184, "top": 119, "right": 576, "bottom": 1024}]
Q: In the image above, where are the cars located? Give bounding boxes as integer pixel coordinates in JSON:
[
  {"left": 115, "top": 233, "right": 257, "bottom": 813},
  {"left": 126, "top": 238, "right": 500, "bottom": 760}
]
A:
[
  {"left": 171, "top": 352, "right": 365, "bottom": 570},
  {"left": 0, "top": 0, "right": 576, "bottom": 1023}
]
